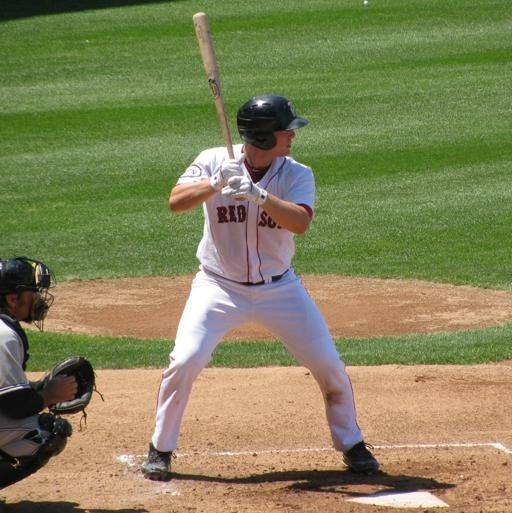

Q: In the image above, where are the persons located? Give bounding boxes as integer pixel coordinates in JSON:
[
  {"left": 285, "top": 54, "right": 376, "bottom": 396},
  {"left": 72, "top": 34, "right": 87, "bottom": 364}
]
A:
[
  {"left": 0, "top": 257, "right": 77, "bottom": 490},
  {"left": 140, "top": 96, "right": 380, "bottom": 481}
]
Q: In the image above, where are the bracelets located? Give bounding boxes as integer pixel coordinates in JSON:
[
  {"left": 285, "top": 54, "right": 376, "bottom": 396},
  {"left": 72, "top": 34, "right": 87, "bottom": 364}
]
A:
[
  {"left": 210, "top": 175, "right": 223, "bottom": 192},
  {"left": 255, "top": 188, "right": 267, "bottom": 205}
]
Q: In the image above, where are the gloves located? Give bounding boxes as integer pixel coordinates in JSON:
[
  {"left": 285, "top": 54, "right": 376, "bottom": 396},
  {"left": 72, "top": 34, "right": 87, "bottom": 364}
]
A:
[
  {"left": 210, "top": 154, "right": 245, "bottom": 191},
  {"left": 222, "top": 177, "right": 267, "bottom": 205}
]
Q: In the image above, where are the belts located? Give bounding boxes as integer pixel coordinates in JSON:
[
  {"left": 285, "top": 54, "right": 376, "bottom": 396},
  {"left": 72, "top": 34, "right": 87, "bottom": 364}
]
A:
[{"left": 240, "top": 268, "right": 288, "bottom": 286}]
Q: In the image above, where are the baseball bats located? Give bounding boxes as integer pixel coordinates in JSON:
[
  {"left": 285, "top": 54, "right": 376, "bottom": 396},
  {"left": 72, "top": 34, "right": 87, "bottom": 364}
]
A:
[{"left": 193, "top": 12, "right": 246, "bottom": 201}]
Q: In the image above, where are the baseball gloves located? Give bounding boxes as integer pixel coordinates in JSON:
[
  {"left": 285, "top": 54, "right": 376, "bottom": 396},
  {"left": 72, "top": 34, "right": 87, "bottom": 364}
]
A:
[{"left": 42, "top": 356, "right": 95, "bottom": 414}]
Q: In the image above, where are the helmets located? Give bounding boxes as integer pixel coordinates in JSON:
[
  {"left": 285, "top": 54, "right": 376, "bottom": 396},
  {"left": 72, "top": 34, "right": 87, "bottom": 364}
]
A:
[
  {"left": 237, "top": 96, "right": 307, "bottom": 150},
  {"left": 0, "top": 256, "right": 56, "bottom": 333}
]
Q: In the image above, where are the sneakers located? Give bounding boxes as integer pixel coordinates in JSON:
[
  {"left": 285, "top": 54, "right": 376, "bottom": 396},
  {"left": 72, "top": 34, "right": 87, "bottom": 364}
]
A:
[
  {"left": 141, "top": 442, "right": 177, "bottom": 481},
  {"left": 342, "top": 440, "right": 379, "bottom": 473}
]
